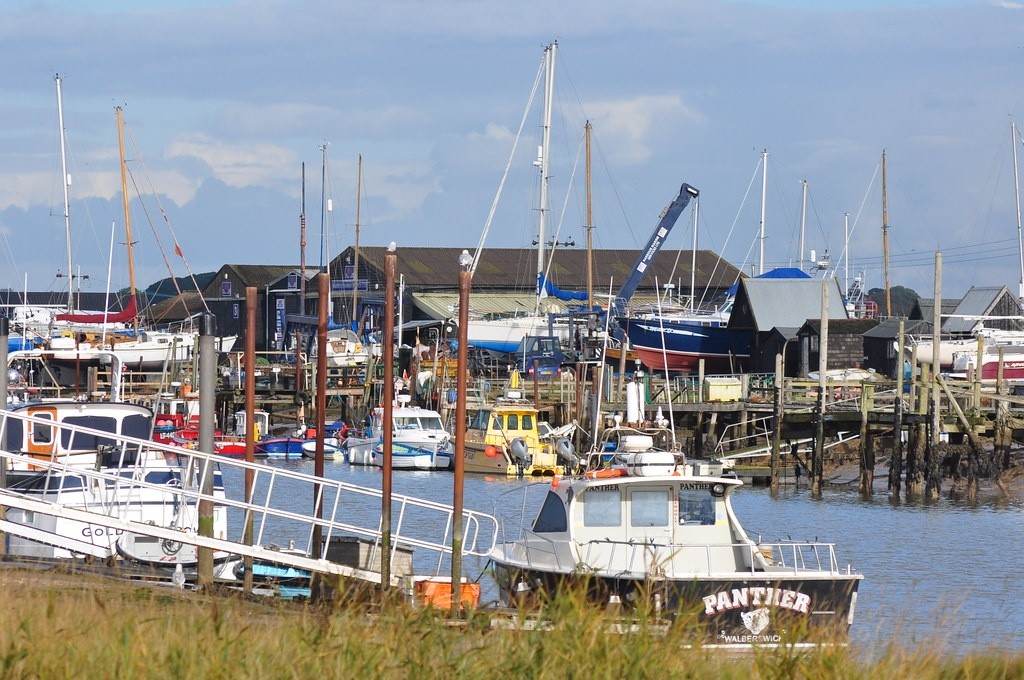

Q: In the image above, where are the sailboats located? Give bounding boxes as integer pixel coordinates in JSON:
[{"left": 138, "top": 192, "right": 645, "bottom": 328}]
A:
[
  {"left": 904, "top": 122, "right": 1024, "bottom": 387},
  {"left": 46, "top": 107, "right": 239, "bottom": 370},
  {"left": 6, "top": 74, "right": 145, "bottom": 349},
  {"left": 451, "top": 42, "right": 604, "bottom": 356},
  {"left": 614, "top": 146, "right": 772, "bottom": 379}
]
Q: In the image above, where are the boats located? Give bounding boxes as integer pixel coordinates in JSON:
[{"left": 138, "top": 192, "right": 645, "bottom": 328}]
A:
[
  {"left": 154, "top": 412, "right": 261, "bottom": 459},
  {"left": 7, "top": 398, "right": 230, "bottom": 574},
  {"left": 348, "top": 394, "right": 455, "bottom": 474},
  {"left": 210, "top": 541, "right": 317, "bottom": 601},
  {"left": 579, "top": 358, "right": 733, "bottom": 483},
  {"left": 459, "top": 364, "right": 564, "bottom": 478},
  {"left": 485, "top": 462, "right": 863, "bottom": 650},
  {"left": 254, "top": 433, "right": 304, "bottom": 460},
  {"left": 301, "top": 439, "right": 344, "bottom": 461}
]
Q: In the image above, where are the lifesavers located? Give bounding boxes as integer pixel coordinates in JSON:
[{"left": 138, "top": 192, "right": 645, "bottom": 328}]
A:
[
  {"left": 294, "top": 391, "right": 311, "bottom": 406},
  {"left": 581, "top": 468, "right": 628, "bottom": 478},
  {"left": 866, "top": 301, "right": 878, "bottom": 316},
  {"left": 62, "top": 331, "right": 73, "bottom": 340},
  {"left": 356, "top": 346, "right": 361, "bottom": 353}
]
